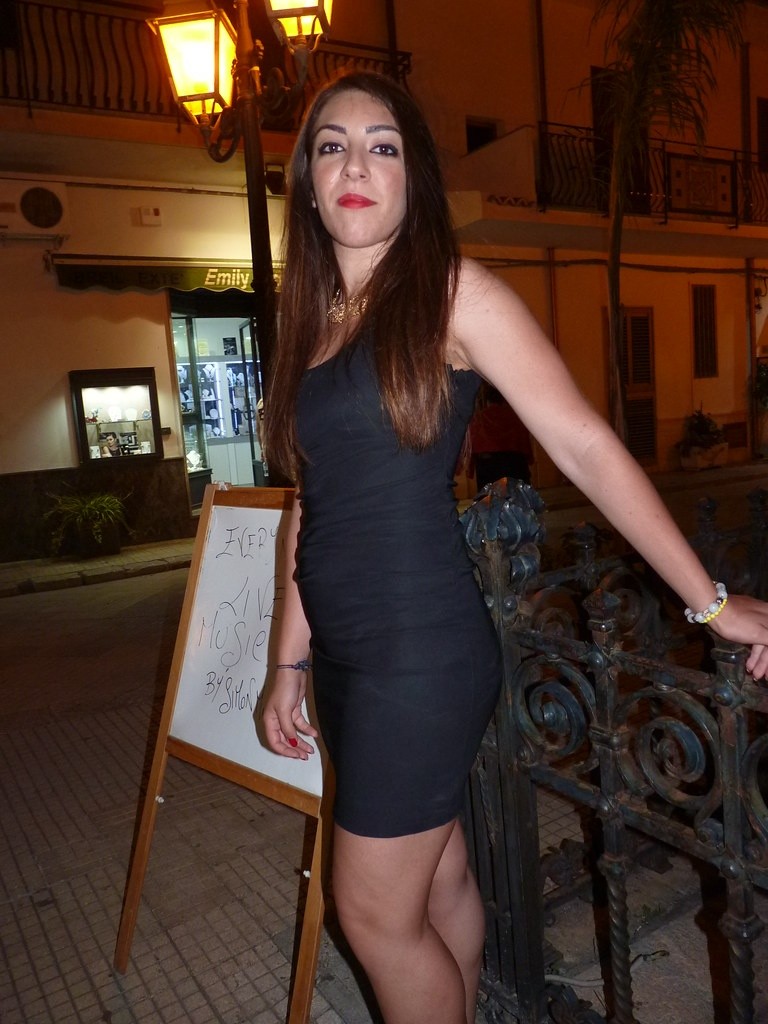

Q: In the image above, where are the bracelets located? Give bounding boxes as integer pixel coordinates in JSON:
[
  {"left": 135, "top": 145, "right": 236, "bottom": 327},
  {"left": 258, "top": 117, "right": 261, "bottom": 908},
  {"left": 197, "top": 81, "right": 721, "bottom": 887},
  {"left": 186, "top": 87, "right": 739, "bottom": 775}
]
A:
[
  {"left": 276, "top": 660, "right": 309, "bottom": 672},
  {"left": 684, "top": 581, "right": 728, "bottom": 624}
]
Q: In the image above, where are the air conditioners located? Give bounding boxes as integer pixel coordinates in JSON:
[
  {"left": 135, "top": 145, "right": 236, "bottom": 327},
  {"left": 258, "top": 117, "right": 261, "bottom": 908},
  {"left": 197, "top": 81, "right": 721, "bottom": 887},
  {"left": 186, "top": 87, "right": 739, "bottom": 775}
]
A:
[{"left": 0, "top": 178, "right": 72, "bottom": 240}]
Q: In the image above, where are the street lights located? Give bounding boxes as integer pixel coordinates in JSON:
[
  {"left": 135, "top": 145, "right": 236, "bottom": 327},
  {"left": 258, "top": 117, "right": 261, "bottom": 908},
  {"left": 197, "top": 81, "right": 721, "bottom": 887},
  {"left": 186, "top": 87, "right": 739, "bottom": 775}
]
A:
[{"left": 145, "top": 0, "right": 333, "bottom": 487}]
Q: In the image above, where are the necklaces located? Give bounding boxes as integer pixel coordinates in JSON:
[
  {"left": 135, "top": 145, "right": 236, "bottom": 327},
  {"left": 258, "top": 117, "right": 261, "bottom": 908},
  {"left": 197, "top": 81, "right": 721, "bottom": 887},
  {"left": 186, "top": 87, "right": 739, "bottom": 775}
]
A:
[
  {"left": 205, "top": 368, "right": 213, "bottom": 378},
  {"left": 327, "top": 289, "right": 370, "bottom": 325}
]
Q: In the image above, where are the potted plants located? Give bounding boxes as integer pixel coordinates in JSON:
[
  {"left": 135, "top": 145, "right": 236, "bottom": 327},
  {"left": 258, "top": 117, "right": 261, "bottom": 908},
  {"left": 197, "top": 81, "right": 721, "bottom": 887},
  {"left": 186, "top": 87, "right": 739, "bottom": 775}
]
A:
[
  {"left": 675, "top": 400, "right": 729, "bottom": 471},
  {"left": 43, "top": 476, "right": 157, "bottom": 562}
]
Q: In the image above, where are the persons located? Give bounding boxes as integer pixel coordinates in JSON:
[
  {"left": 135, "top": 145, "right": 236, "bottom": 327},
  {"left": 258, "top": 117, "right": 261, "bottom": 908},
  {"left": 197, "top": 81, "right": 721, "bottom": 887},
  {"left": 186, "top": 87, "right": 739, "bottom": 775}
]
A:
[
  {"left": 252, "top": 72, "right": 768, "bottom": 1024},
  {"left": 104, "top": 432, "right": 122, "bottom": 457}
]
